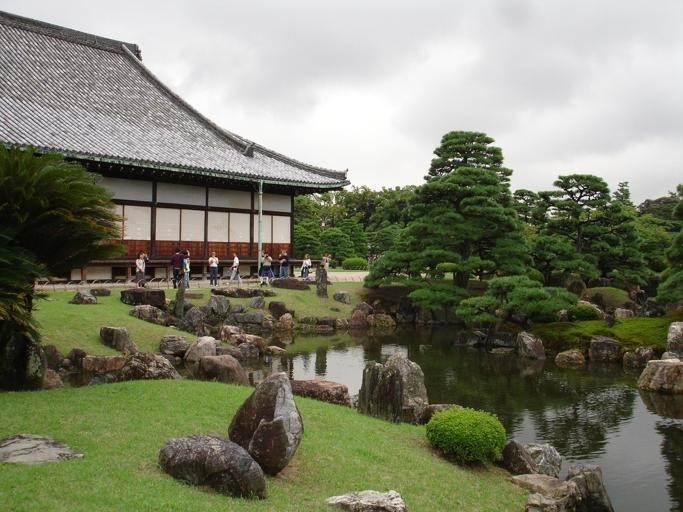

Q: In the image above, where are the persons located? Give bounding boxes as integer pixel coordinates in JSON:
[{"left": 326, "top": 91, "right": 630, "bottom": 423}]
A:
[{"left": 134, "top": 247, "right": 332, "bottom": 287}]
